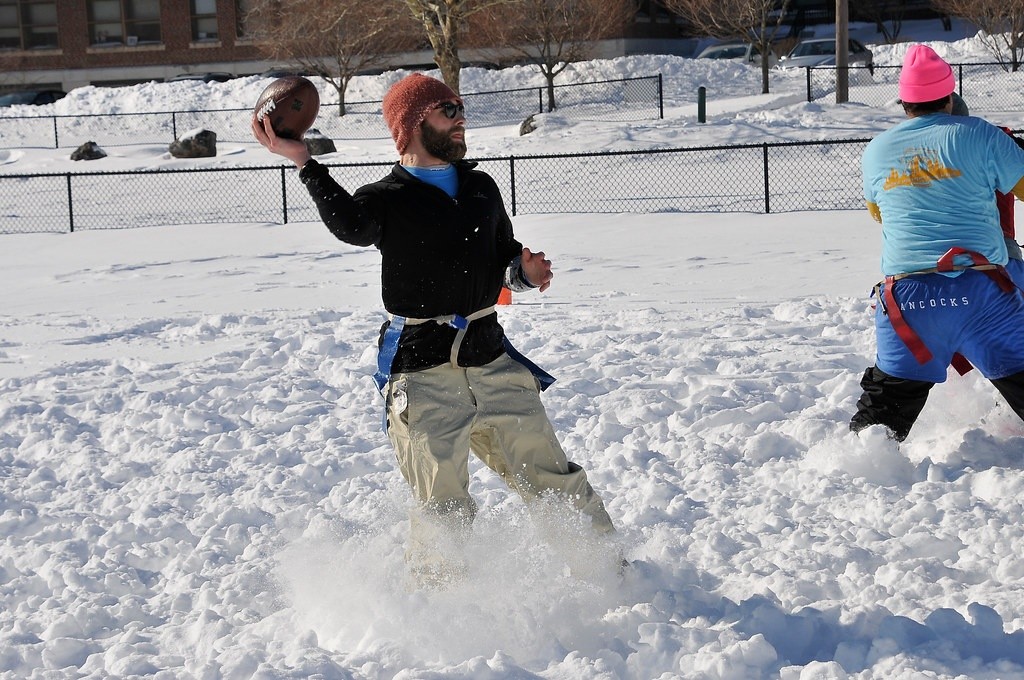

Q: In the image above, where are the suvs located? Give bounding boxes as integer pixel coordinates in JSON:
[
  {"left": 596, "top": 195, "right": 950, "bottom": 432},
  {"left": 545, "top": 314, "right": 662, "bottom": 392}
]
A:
[{"left": 165, "top": 72, "right": 236, "bottom": 84}]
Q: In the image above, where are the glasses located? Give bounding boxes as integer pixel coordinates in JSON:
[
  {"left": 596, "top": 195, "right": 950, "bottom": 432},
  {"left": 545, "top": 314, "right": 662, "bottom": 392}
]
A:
[{"left": 433, "top": 102, "right": 465, "bottom": 118}]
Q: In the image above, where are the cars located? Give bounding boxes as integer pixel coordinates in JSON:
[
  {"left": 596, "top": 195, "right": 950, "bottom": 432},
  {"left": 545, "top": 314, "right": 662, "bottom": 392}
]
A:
[
  {"left": 779, "top": 37, "right": 874, "bottom": 77},
  {"left": 260, "top": 67, "right": 329, "bottom": 78},
  {"left": 0, "top": 88, "right": 67, "bottom": 107},
  {"left": 696, "top": 42, "right": 778, "bottom": 70}
]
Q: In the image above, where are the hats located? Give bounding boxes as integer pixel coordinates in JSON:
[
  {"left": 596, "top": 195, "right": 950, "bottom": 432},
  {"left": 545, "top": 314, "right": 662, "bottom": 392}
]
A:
[
  {"left": 384, "top": 73, "right": 463, "bottom": 155},
  {"left": 899, "top": 44, "right": 955, "bottom": 103}
]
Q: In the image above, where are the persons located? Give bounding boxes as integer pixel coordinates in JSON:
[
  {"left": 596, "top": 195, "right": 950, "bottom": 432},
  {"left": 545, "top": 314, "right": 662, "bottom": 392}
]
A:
[
  {"left": 252, "top": 74, "right": 628, "bottom": 578},
  {"left": 847, "top": 44, "right": 1024, "bottom": 450}
]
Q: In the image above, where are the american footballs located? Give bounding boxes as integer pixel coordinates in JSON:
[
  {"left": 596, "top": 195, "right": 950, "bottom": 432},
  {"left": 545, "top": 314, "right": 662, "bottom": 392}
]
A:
[{"left": 255, "top": 74, "right": 320, "bottom": 138}]
